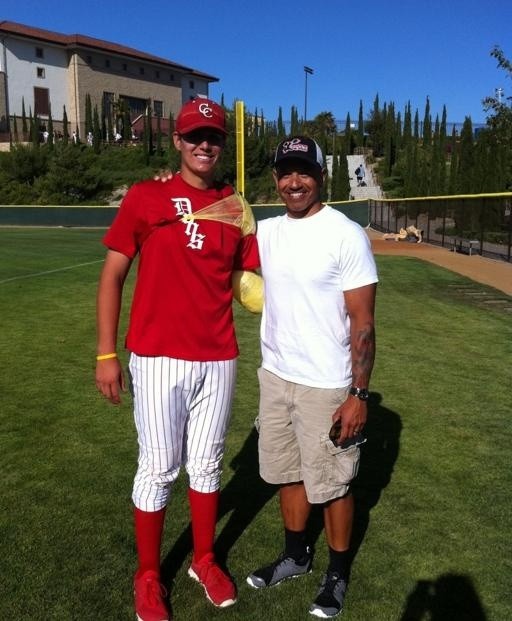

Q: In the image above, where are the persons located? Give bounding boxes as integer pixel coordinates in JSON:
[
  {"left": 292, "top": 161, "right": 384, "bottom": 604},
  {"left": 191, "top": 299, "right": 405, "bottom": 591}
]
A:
[
  {"left": 93, "top": 98, "right": 263, "bottom": 621},
  {"left": 38, "top": 122, "right": 123, "bottom": 148},
  {"left": 153, "top": 135, "right": 379, "bottom": 619},
  {"left": 356, "top": 164, "right": 365, "bottom": 186}
]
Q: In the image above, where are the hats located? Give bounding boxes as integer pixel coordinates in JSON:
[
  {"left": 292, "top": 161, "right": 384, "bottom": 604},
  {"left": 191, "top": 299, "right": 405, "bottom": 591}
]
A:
[
  {"left": 175, "top": 97, "right": 228, "bottom": 138},
  {"left": 273, "top": 136, "right": 324, "bottom": 172}
]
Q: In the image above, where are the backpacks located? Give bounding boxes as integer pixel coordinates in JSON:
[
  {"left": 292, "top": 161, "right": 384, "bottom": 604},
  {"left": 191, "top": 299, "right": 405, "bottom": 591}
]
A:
[{"left": 355, "top": 167, "right": 360, "bottom": 176}]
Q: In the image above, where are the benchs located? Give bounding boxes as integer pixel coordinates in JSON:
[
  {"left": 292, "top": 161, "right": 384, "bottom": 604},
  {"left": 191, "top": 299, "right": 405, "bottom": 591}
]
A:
[{"left": 448, "top": 233, "right": 480, "bottom": 254}]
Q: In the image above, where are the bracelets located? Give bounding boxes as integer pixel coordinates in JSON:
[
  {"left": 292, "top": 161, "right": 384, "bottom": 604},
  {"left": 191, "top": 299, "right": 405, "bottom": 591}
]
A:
[{"left": 95, "top": 352, "right": 119, "bottom": 360}]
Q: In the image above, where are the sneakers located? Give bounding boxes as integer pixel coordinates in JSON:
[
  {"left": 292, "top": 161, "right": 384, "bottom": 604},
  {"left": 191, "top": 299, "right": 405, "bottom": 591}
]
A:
[
  {"left": 133, "top": 568, "right": 170, "bottom": 621},
  {"left": 187, "top": 552, "right": 239, "bottom": 610},
  {"left": 308, "top": 563, "right": 351, "bottom": 619},
  {"left": 246, "top": 546, "right": 313, "bottom": 590}
]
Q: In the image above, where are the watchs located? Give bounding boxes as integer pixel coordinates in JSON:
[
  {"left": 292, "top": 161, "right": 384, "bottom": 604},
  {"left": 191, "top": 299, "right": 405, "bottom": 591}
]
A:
[{"left": 348, "top": 386, "right": 370, "bottom": 401}]
